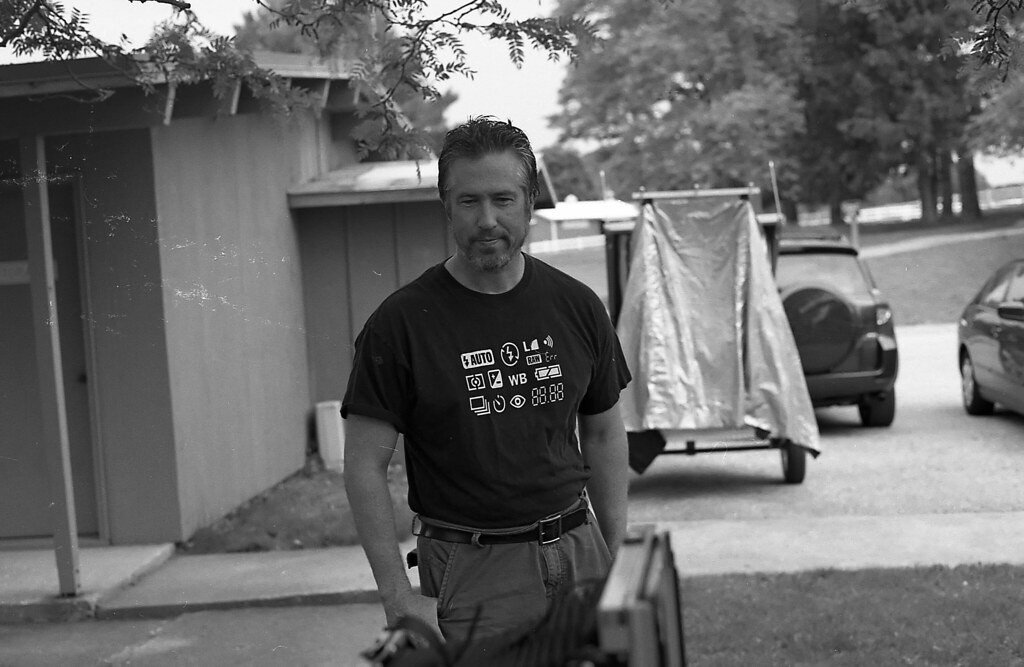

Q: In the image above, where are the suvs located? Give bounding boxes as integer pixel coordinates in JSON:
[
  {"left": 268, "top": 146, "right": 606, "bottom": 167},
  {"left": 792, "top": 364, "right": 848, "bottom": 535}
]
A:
[{"left": 773, "top": 235, "right": 899, "bottom": 427}]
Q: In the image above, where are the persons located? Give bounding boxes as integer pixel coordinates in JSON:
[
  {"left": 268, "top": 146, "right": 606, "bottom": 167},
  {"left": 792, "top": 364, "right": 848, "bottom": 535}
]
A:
[{"left": 340, "top": 113, "right": 632, "bottom": 667}]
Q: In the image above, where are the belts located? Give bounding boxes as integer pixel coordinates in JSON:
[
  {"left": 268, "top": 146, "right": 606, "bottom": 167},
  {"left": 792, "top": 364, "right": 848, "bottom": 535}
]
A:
[{"left": 420, "top": 491, "right": 597, "bottom": 546}]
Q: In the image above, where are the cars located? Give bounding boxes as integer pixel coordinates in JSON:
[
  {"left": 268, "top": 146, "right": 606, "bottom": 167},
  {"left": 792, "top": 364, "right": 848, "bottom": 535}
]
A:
[{"left": 956, "top": 257, "right": 1024, "bottom": 419}]
